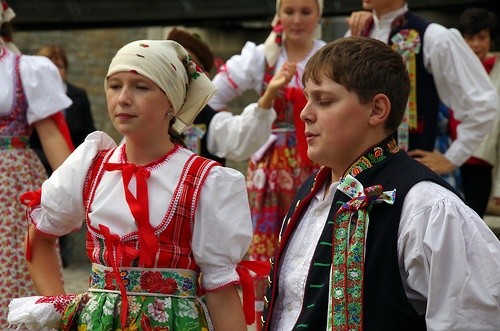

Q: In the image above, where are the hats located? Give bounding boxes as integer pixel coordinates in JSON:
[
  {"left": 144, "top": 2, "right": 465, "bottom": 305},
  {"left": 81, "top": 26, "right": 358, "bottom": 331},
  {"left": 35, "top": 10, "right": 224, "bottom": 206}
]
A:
[{"left": 167, "top": 28, "right": 213, "bottom": 74}]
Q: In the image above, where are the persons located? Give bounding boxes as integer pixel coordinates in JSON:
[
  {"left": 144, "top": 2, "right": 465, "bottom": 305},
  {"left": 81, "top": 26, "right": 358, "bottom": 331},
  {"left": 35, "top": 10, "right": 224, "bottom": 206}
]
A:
[{"left": 0, "top": 0, "right": 500, "bottom": 331}]
